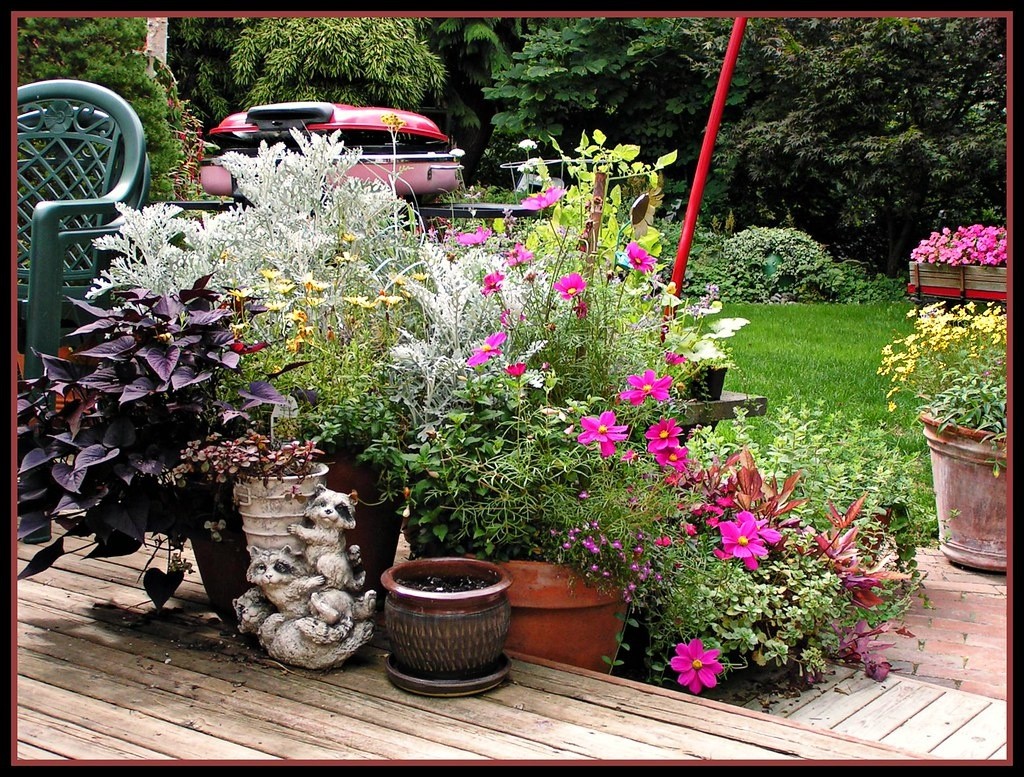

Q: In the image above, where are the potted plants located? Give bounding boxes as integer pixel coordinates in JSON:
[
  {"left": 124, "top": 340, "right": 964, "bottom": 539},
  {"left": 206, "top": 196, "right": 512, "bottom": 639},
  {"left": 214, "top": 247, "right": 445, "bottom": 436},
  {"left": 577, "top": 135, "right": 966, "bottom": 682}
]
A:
[
  {"left": 686, "top": 341, "right": 756, "bottom": 424},
  {"left": 16, "top": 125, "right": 426, "bottom": 633},
  {"left": 163, "top": 429, "right": 376, "bottom": 668},
  {"left": 809, "top": 410, "right": 923, "bottom": 569}
]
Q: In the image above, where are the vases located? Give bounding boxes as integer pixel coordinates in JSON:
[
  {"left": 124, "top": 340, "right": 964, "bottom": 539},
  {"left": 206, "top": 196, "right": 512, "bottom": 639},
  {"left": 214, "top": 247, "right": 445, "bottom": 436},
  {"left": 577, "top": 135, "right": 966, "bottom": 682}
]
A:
[
  {"left": 920, "top": 412, "right": 1007, "bottom": 572},
  {"left": 460, "top": 549, "right": 625, "bottom": 674},
  {"left": 380, "top": 557, "right": 513, "bottom": 697}
]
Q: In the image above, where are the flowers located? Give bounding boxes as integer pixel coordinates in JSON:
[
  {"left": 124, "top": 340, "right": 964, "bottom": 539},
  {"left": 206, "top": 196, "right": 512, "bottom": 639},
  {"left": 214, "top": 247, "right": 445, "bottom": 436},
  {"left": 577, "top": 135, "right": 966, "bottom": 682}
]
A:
[
  {"left": 911, "top": 224, "right": 1007, "bottom": 267},
  {"left": 411, "top": 180, "right": 912, "bottom": 696},
  {"left": 877, "top": 300, "right": 1008, "bottom": 478}
]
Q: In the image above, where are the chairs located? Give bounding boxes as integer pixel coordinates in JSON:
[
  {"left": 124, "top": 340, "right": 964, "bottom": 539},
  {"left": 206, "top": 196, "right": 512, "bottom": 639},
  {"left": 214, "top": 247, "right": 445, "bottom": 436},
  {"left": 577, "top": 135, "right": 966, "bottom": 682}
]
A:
[{"left": 16, "top": 77, "right": 152, "bottom": 380}]
